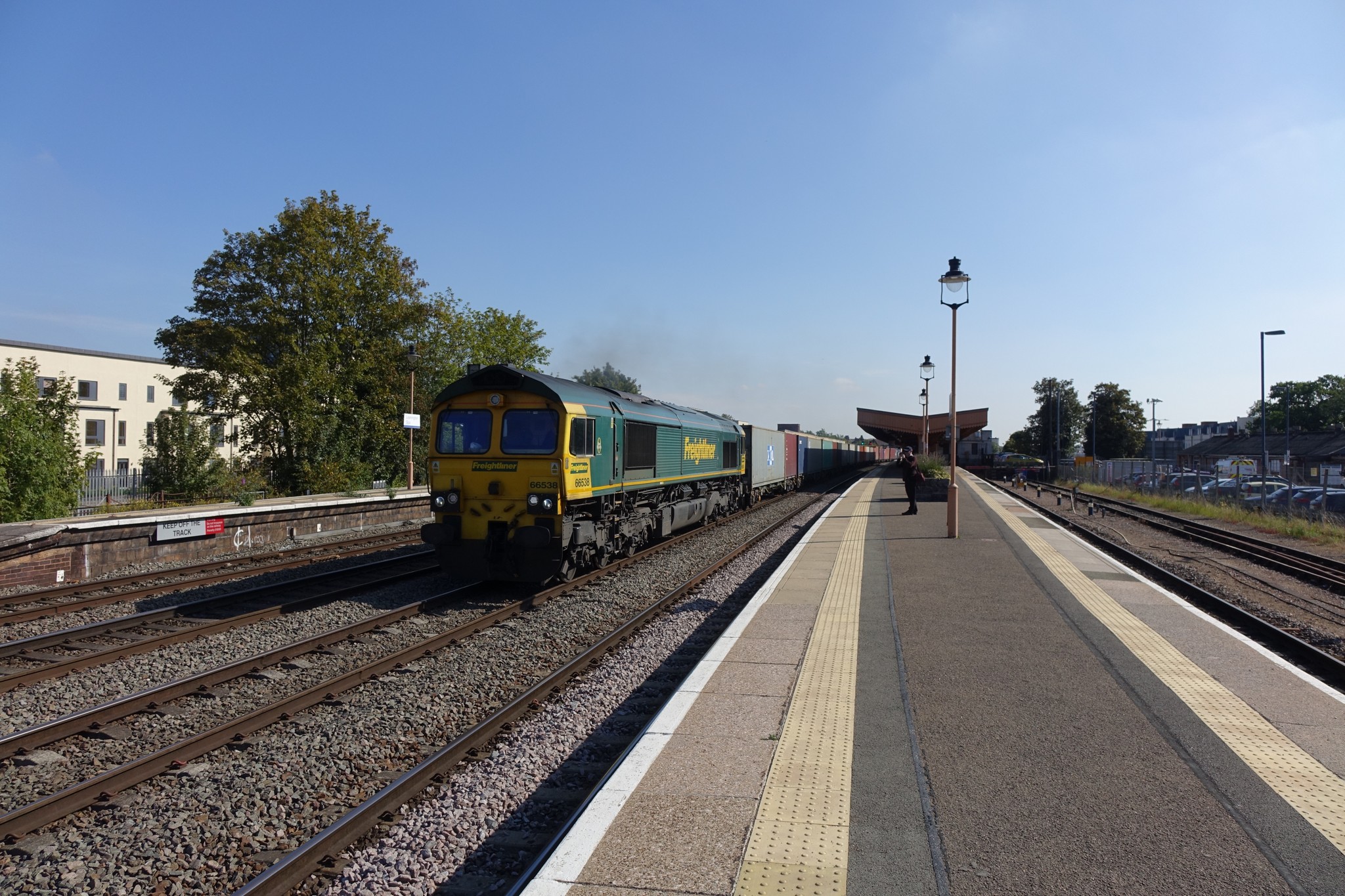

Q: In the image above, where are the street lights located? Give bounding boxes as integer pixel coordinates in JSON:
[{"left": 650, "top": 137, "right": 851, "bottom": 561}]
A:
[
  {"left": 937, "top": 257, "right": 972, "bottom": 538},
  {"left": 1274, "top": 386, "right": 1293, "bottom": 519},
  {"left": 1260, "top": 330, "right": 1286, "bottom": 511},
  {"left": 918, "top": 354, "right": 935, "bottom": 457},
  {"left": 1146, "top": 398, "right": 1163, "bottom": 492},
  {"left": 1092, "top": 391, "right": 1106, "bottom": 485},
  {"left": 918, "top": 388, "right": 929, "bottom": 454}
]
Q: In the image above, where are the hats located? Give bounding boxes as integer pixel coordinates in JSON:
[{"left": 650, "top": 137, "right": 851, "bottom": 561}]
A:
[{"left": 903, "top": 446, "right": 913, "bottom": 451}]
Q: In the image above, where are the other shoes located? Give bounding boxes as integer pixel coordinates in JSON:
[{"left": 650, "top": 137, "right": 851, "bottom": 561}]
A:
[{"left": 901, "top": 509, "right": 916, "bottom": 515}]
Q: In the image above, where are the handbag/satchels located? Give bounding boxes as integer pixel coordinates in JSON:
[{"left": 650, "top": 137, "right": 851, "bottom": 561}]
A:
[{"left": 916, "top": 471, "right": 925, "bottom": 486}]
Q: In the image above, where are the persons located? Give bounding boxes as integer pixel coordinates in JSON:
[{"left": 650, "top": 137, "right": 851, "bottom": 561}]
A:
[{"left": 895, "top": 446, "right": 918, "bottom": 516}]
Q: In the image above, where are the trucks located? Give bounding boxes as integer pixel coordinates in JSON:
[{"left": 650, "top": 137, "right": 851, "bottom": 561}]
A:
[{"left": 1214, "top": 455, "right": 1257, "bottom": 480}]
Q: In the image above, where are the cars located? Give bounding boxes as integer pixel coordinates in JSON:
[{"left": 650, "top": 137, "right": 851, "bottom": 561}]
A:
[
  {"left": 994, "top": 452, "right": 1026, "bottom": 466},
  {"left": 1309, "top": 492, "right": 1345, "bottom": 518},
  {"left": 1115, "top": 471, "right": 1181, "bottom": 492},
  {"left": 1169, "top": 474, "right": 1222, "bottom": 498},
  {"left": 999, "top": 454, "right": 1044, "bottom": 467},
  {"left": 1242, "top": 486, "right": 1282, "bottom": 513},
  {"left": 1269, "top": 487, "right": 1325, "bottom": 517},
  {"left": 1239, "top": 482, "right": 1289, "bottom": 508},
  {"left": 1184, "top": 473, "right": 1297, "bottom": 504},
  {"left": 1291, "top": 489, "right": 1345, "bottom": 517}
]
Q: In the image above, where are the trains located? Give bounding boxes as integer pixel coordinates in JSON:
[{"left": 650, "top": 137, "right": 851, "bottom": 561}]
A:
[{"left": 420, "top": 362, "right": 899, "bottom": 586}]
